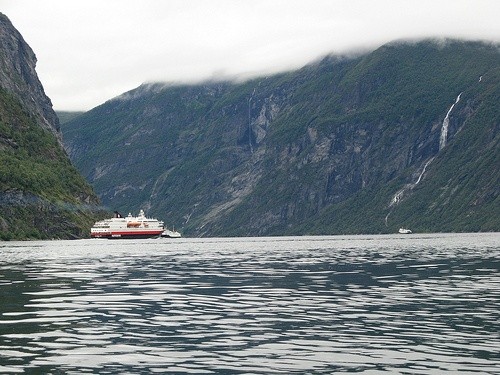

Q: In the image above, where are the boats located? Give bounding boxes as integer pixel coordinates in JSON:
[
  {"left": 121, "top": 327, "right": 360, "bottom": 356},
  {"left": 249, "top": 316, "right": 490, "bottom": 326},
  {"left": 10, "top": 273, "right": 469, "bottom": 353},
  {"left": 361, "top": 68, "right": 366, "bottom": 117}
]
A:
[
  {"left": 91, "top": 209, "right": 164, "bottom": 239},
  {"left": 399, "top": 228, "right": 412, "bottom": 234},
  {"left": 160, "top": 224, "right": 182, "bottom": 238}
]
